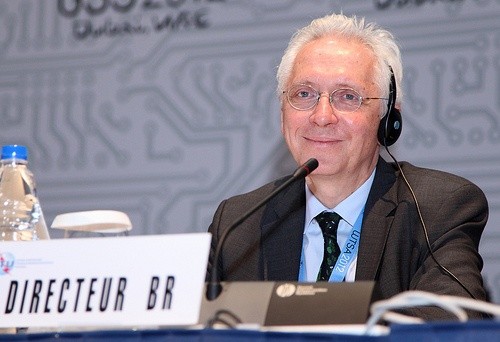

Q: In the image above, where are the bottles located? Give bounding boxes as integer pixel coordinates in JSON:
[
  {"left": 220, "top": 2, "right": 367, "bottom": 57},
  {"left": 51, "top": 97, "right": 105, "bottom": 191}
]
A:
[{"left": 1, "top": 144, "right": 41, "bottom": 242}]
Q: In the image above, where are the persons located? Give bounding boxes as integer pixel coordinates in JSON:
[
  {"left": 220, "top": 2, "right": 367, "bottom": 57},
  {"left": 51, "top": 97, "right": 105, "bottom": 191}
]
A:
[{"left": 203, "top": 8, "right": 490, "bottom": 327}]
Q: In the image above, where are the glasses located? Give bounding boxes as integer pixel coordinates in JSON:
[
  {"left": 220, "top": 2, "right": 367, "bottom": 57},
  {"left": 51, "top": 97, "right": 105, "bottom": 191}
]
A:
[{"left": 282, "top": 84, "right": 387, "bottom": 113}]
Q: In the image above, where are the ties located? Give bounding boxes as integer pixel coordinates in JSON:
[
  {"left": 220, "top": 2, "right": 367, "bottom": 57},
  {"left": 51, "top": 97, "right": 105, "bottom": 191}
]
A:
[{"left": 315, "top": 212, "right": 345, "bottom": 281}]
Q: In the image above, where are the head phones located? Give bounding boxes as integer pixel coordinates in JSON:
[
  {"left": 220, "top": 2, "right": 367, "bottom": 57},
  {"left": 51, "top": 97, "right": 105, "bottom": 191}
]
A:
[{"left": 377, "top": 65, "right": 402, "bottom": 146}]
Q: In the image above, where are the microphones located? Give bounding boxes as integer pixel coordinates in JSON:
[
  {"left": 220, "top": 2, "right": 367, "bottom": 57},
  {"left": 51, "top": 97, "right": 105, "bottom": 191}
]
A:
[{"left": 208, "top": 159, "right": 318, "bottom": 298}]
